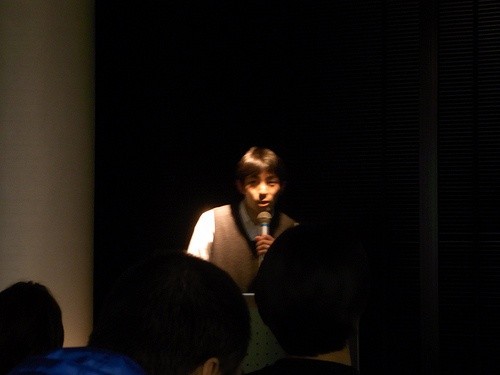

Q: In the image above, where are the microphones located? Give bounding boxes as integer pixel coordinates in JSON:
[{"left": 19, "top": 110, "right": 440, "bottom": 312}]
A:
[{"left": 256, "top": 211, "right": 272, "bottom": 268}]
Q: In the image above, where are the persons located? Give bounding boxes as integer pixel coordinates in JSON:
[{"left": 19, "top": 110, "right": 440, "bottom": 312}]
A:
[
  {"left": 186, "top": 146, "right": 301, "bottom": 293},
  {"left": 0, "top": 215, "right": 375, "bottom": 375}
]
